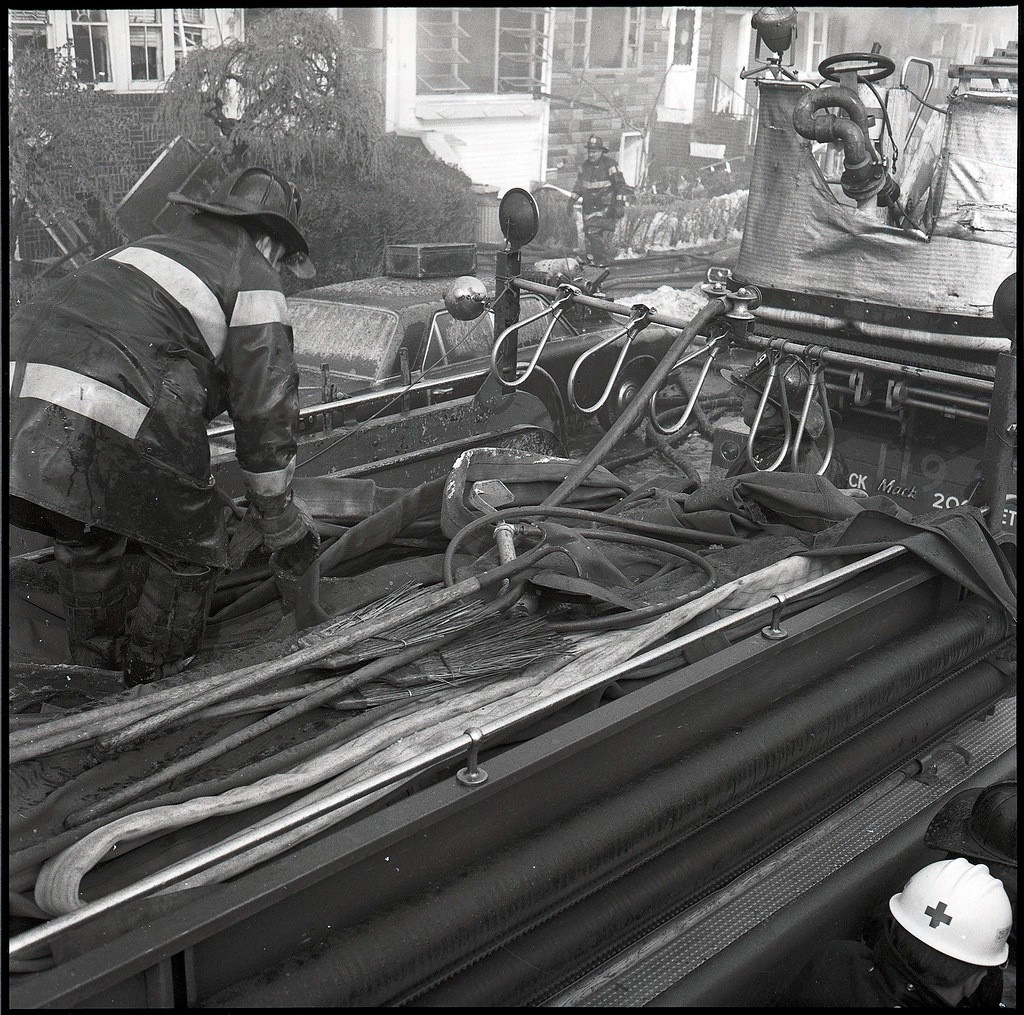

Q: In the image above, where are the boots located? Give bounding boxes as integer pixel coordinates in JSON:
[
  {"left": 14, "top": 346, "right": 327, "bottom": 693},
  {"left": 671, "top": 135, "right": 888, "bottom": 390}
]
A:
[
  {"left": 125, "top": 557, "right": 213, "bottom": 689},
  {"left": 54, "top": 537, "right": 133, "bottom": 667}
]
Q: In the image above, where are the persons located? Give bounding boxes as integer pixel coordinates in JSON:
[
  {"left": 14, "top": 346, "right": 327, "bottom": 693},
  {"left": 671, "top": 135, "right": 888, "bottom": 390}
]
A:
[
  {"left": 722, "top": 353, "right": 829, "bottom": 482},
  {"left": 568, "top": 134, "right": 625, "bottom": 264},
  {"left": 791, "top": 858, "right": 1014, "bottom": 1008},
  {"left": 10, "top": 168, "right": 320, "bottom": 688}
]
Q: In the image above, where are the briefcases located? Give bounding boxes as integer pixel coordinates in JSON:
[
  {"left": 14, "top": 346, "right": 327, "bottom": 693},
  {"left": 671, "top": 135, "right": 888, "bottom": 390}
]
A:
[{"left": 386, "top": 241, "right": 478, "bottom": 278}]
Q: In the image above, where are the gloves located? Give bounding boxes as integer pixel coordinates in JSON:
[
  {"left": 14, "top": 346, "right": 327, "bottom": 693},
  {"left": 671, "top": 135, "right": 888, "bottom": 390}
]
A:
[
  {"left": 566, "top": 204, "right": 573, "bottom": 217},
  {"left": 255, "top": 487, "right": 309, "bottom": 549},
  {"left": 614, "top": 206, "right": 625, "bottom": 218}
]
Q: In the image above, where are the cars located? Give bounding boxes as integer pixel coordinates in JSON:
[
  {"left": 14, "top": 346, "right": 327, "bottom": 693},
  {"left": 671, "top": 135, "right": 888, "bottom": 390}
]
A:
[{"left": 204, "top": 272, "right": 677, "bottom": 434}]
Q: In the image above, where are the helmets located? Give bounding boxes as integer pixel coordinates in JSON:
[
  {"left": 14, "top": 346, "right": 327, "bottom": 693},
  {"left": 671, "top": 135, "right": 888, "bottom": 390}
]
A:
[
  {"left": 584, "top": 134, "right": 609, "bottom": 154},
  {"left": 170, "top": 165, "right": 317, "bottom": 279},
  {"left": 888, "top": 858, "right": 1013, "bottom": 965},
  {"left": 720, "top": 336, "right": 826, "bottom": 437}
]
